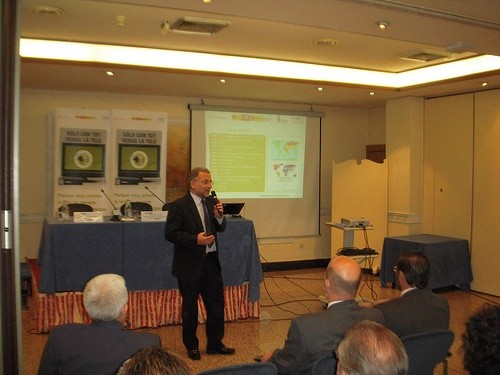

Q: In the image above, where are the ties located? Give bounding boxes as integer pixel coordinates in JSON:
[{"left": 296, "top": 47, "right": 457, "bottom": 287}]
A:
[{"left": 201, "top": 199, "right": 215, "bottom": 250}]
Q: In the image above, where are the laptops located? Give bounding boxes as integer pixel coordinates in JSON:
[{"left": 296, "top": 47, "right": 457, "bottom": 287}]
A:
[{"left": 221, "top": 202, "right": 245, "bottom": 216}]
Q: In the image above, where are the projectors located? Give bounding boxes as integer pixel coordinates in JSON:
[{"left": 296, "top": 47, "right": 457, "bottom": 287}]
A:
[{"left": 340, "top": 216, "right": 370, "bottom": 227}]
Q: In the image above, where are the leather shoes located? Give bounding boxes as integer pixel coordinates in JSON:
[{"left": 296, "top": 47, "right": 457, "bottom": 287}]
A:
[
  {"left": 207, "top": 340, "right": 235, "bottom": 356},
  {"left": 188, "top": 349, "right": 201, "bottom": 361}
]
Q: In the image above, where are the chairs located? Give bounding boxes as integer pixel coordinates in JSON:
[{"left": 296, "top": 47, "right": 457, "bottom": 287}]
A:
[
  {"left": 195, "top": 328, "right": 455, "bottom": 375},
  {"left": 121, "top": 202, "right": 152, "bottom": 217},
  {"left": 58, "top": 204, "right": 93, "bottom": 216}
]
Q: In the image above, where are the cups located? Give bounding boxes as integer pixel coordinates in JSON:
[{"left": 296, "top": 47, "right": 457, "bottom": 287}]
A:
[
  {"left": 133, "top": 210, "right": 139, "bottom": 219},
  {"left": 56, "top": 212, "right": 63, "bottom": 221}
]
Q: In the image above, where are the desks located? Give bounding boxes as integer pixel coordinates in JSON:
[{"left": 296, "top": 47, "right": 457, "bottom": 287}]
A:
[
  {"left": 380, "top": 234, "right": 473, "bottom": 291},
  {"left": 323, "top": 222, "right": 374, "bottom": 247},
  {"left": 333, "top": 254, "right": 379, "bottom": 300},
  {"left": 39, "top": 214, "right": 263, "bottom": 304}
]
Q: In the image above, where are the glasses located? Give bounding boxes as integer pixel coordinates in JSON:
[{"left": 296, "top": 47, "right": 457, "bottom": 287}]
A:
[
  {"left": 393, "top": 266, "right": 399, "bottom": 272},
  {"left": 194, "top": 179, "right": 215, "bottom": 186}
]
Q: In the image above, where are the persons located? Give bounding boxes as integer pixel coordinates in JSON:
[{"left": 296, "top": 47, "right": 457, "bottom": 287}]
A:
[
  {"left": 335, "top": 321, "right": 409, "bottom": 375},
  {"left": 458, "top": 304, "right": 500, "bottom": 375},
  {"left": 37, "top": 274, "right": 162, "bottom": 375},
  {"left": 115, "top": 348, "right": 196, "bottom": 375},
  {"left": 261, "top": 255, "right": 385, "bottom": 375},
  {"left": 164, "top": 167, "right": 236, "bottom": 361},
  {"left": 373, "top": 252, "right": 449, "bottom": 340}
]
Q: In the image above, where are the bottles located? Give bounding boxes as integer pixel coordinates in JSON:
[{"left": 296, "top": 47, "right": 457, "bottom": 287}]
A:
[
  {"left": 62, "top": 200, "right": 69, "bottom": 219},
  {"left": 124, "top": 201, "right": 133, "bottom": 217}
]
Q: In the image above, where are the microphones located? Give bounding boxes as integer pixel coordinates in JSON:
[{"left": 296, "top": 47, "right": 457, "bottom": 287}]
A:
[
  {"left": 211, "top": 190, "right": 222, "bottom": 216},
  {"left": 101, "top": 189, "right": 115, "bottom": 209},
  {"left": 145, "top": 186, "right": 165, "bottom": 205}
]
